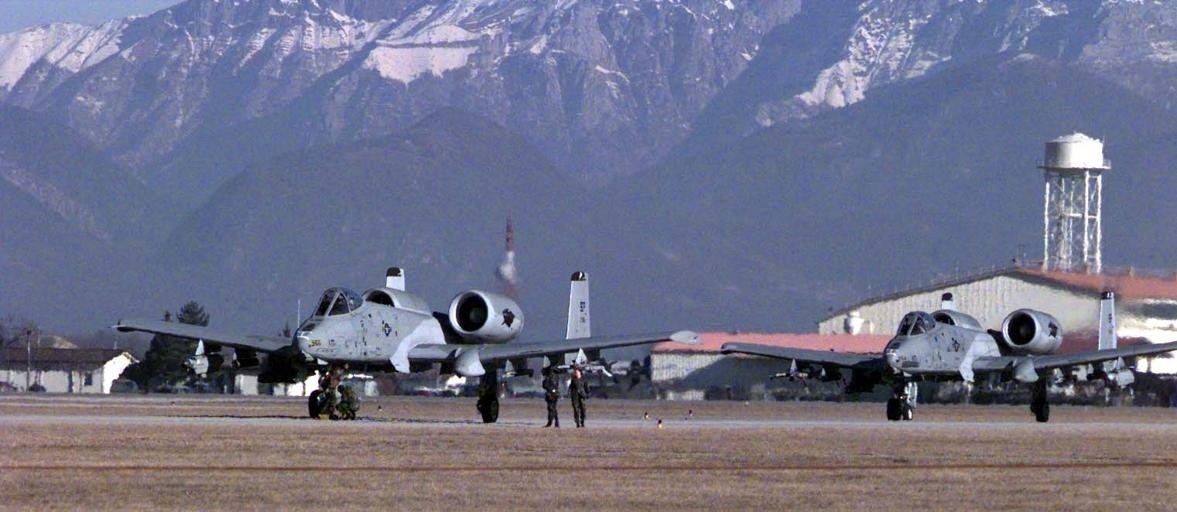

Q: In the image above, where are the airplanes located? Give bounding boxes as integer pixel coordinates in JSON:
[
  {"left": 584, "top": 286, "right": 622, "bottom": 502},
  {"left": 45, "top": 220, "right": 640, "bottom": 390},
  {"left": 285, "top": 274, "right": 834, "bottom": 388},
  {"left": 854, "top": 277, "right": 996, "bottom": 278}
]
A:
[
  {"left": 105, "top": 261, "right": 706, "bottom": 423},
  {"left": 714, "top": 288, "right": 1176, "bottom": 423}
]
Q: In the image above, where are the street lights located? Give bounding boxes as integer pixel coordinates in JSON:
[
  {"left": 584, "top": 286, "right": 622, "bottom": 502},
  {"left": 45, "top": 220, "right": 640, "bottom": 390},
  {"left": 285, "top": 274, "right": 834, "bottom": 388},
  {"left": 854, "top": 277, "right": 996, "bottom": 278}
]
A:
[{"left": 24, "top": 328, "right": 34, "bottom": 391}]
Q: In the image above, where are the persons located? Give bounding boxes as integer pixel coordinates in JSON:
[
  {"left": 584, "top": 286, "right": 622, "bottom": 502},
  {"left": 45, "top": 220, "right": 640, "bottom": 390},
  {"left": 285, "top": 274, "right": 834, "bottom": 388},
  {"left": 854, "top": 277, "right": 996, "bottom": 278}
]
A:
[
  {"left": 567, "top": 369, "right": 592, "bottom": 429},
  {"left": 310, "top": 361, "right": 350, "bottom": 421},
  {"left": 335, "top": 383, "right": 361, "bottom": 421},
  {"left": 542, "top": 373, "right": 559, "bottom": 429}
]
